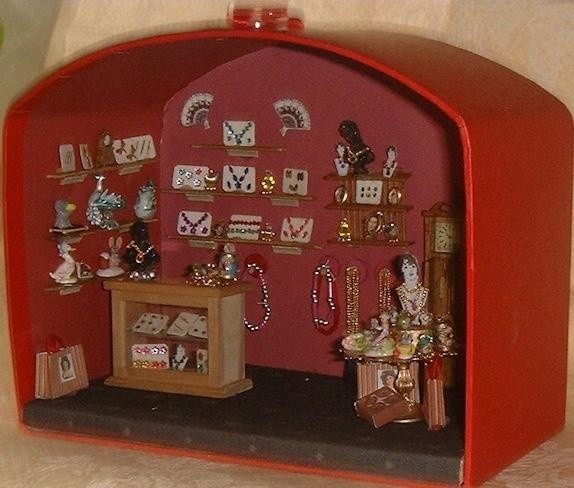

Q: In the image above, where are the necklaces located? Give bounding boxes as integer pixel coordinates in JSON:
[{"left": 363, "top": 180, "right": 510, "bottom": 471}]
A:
[
  {"left": 173, "top": 355, "right": 187, "bottom": 370},
  {"left": 181, "top": 212, "right": 207, "bottom": 234},
  {"left": 286, "top": 218, "right": 313, "bottom": 239},
  {"left": 307, "top": 261, "right": 391, "bottom": 338},
  {"left": 225, "top": 226, "right": 260, "bottom": 235},
  {"left": 129, "top": 241, "right": 155, "bottom": 265},
  {"left": 227, "top": 219, "right": 261, "bottom": 226},
  {"left": 396, "top": 282, "right": 428, "bottom": 318},
  {"left": 226, "top": 166, "right": 249, "bottom": 189},
  {"left": 223, "top": 121, "right": 252, "bottom": 144},
  {"left": 236, "top": 263, "right": 271, "bottom": 332}
]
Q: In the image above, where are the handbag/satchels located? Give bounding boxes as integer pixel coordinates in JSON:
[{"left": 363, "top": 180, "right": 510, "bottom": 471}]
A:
[{"left": 33, "top": 333, "right": 92, "bottom": 401}]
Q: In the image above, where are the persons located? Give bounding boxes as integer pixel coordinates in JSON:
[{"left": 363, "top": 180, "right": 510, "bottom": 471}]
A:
[
  {"left": 380, "top": 371, "right": 398, "bottom": 388},
  {"left": 332, "top": 120, "right": 399, "bottom": 180},
  {"left": 57, "top": 355, "right": 74, "bottom": 380},
  {"left": 392, "top": 252, "right": 430, "bottom": 314},
  {"left": 367, "top": 216, "right": 377, "bottom": 231},
  {"left": 122, "top": 220, "right": 160, "bottom": 273}
]
